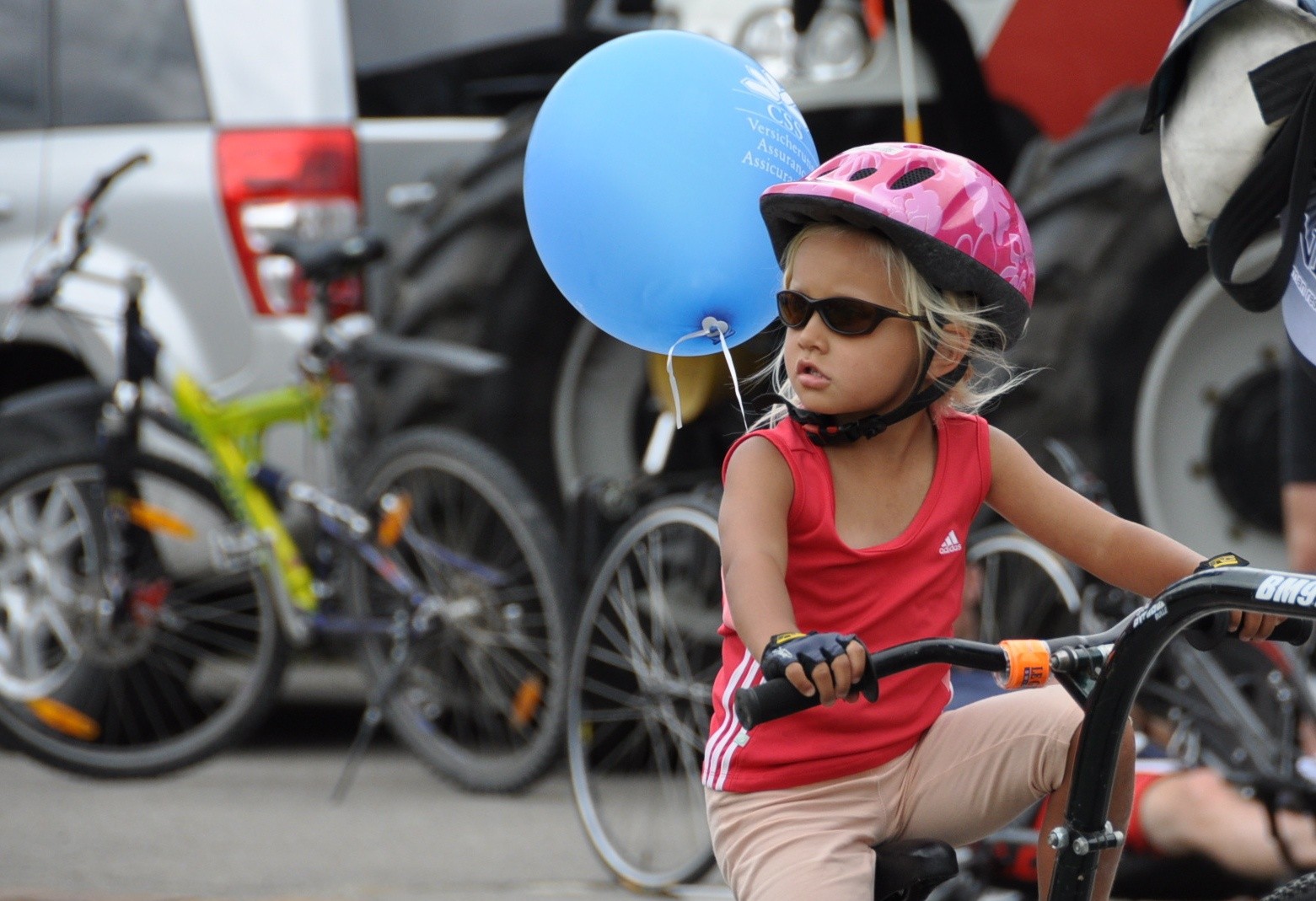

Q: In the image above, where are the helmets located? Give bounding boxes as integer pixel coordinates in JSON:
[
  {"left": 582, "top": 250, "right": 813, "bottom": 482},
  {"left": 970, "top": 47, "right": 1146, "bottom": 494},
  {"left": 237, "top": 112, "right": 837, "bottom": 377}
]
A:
[{"left": 760, "top": 142, "right": 1034, "bottom": 349}]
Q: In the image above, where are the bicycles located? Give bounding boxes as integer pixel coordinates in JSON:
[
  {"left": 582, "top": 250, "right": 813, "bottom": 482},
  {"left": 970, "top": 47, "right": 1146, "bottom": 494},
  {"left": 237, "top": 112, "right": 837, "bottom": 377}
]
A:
[
  {"left": 564, "top": 360, "right": 1316, "bottom": 901},
  {"left": 0, "top": 146, "right": 582, "bottom": 800},
  {"left": 733, "top": 561, "right": 1316, "bottom": 901}
]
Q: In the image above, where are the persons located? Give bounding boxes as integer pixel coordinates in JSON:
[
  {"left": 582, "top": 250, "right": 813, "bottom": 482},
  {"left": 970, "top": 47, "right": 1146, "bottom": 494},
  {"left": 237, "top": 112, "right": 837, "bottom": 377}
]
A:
[
  {"left": 943, "top": 549, "right": 1316, "bottom": 888},
  {"left": 1139, "top": 0, "right": 1316, "bottom": 757},
  {"left": 705, "top": 144, "right": 1288, "bottom": 901}
]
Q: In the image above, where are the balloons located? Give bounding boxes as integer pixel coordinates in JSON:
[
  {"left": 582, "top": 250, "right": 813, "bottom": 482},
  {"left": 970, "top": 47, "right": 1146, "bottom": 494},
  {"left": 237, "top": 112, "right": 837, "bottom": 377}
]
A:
[{"left": 521, "top": 26, "right": 821, "bottom": 362}]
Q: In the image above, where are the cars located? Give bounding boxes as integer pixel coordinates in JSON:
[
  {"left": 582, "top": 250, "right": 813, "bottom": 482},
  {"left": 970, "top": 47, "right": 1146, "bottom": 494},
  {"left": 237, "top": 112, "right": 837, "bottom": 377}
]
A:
[{"left": 0, "top": 0, "right": 957, "bottom": 743}]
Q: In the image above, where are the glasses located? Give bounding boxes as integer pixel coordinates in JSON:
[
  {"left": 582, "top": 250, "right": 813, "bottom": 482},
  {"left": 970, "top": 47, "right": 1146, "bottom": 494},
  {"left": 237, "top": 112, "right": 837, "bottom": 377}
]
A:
[{"left": 775, "top": 290, "right": 941, "bottom": 338}]
